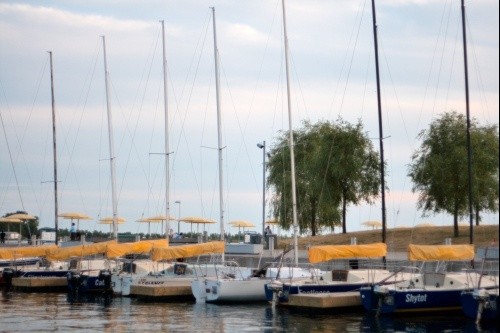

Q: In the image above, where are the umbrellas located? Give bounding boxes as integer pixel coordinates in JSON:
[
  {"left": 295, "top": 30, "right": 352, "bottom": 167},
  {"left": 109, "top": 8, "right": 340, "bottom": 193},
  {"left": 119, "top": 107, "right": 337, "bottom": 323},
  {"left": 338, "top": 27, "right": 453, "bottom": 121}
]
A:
[
  {"left": 226, "top": 219, "right": 256, "bottom": 243},
  {"left": 176, "top": 216, "right": 218, "bottom": 238},
  {"left": 0, "top": 214, "right": 37, "bottom": 233},
  {"left": 97, "top": 216, "right": 127, "bottom": 238},
  {"left": 135, "top": 214, "right": 176, "bottom": 236},
  {"left": 265, "top": 219, "right": 279, "bottom": 233},
  {"left": 58, "top": 211, "right": 92, "bottom": 229},
  {"left": 361, "top": 220, "right": 382, "bottom": 230}
]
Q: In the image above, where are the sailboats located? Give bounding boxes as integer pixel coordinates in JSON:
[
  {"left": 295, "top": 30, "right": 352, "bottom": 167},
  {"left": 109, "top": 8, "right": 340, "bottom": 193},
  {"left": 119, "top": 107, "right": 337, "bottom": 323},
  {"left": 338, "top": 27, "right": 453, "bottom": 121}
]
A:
[
  {"left": 0, "top": 0, "right": 252, "bottom": 299},
  {"left": 260, "top": 0, "right": 425, "bottom": 318},
  {"left": 187, "top": 7, "right": 330, "bottom": 305},
  {"left": 357, "top": 1, "right": 500, "bottom": 333}
]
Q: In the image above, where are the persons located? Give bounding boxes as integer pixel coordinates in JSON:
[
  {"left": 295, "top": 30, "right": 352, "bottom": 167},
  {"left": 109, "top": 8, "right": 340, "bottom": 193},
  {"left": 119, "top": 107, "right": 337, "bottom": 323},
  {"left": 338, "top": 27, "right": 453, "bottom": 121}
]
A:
[
  {"left": 0, "top": 231, "right": 6, "bottom": 244},
  {"left": 70, "top": 223, "right": 77, "bottom": 241},
  {"left": 264, "top": 226, "right": 272, "bottom": 234}
]
